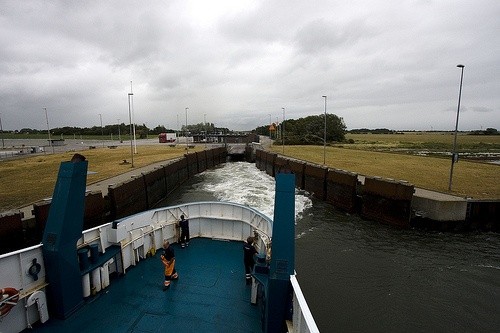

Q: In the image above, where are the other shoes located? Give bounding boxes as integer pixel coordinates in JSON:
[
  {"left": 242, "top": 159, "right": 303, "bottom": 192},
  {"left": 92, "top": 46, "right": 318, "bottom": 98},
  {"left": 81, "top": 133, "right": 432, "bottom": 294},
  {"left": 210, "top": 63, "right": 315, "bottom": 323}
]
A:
[
  {"left": 181, "top": 244, "right": 185, "bottom": 248},
  {"left": 186, "top": 242, "right": 190, "bottom": 247}
]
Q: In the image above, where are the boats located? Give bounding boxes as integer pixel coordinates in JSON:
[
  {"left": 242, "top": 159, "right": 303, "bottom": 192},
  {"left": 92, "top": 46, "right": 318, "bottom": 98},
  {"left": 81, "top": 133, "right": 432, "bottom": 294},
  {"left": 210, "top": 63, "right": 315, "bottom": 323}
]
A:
[{"left": 1, "top": 152, "right": 321, "bottom": 333}]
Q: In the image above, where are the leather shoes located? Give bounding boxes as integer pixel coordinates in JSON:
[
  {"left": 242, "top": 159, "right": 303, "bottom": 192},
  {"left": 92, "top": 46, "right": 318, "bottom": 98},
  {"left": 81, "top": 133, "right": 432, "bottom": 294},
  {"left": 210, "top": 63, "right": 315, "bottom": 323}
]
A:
[
  {"left": 164, "top": 284, "right": 170, "bottom": 291},
  {"left": 171, "top": 276, "right": 178, "bottom": 280}
]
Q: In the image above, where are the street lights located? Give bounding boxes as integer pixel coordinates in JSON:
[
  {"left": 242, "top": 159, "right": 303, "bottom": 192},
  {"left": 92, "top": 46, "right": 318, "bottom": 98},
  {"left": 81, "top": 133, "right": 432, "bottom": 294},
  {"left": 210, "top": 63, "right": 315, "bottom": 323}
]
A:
[
  {"left": 99, "top": 60, "right": 469, "bottom": 191},
  {"left": 41, "top": 106, "right": 55, "bottom": 154}
]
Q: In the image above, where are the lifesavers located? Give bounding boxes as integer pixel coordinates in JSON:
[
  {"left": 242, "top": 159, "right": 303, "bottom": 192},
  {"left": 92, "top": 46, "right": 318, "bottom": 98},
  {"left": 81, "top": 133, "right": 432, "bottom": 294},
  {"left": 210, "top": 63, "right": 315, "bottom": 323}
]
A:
[{"left": 0, "top": 287, "right": 19, "bottom": 317}]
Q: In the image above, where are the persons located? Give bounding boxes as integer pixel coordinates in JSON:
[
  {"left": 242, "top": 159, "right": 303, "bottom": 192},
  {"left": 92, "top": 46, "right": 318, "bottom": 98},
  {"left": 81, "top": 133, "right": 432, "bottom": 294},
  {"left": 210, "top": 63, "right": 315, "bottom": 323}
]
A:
[
  {"left": 179, "top": 214, "right": 189, "bottom": 247},
  {"left": 242, "top": 236, "right": 256, "bottom": 285},
  {"left": 160, "top": 240, "right": 178, "bottom": 291}
]
